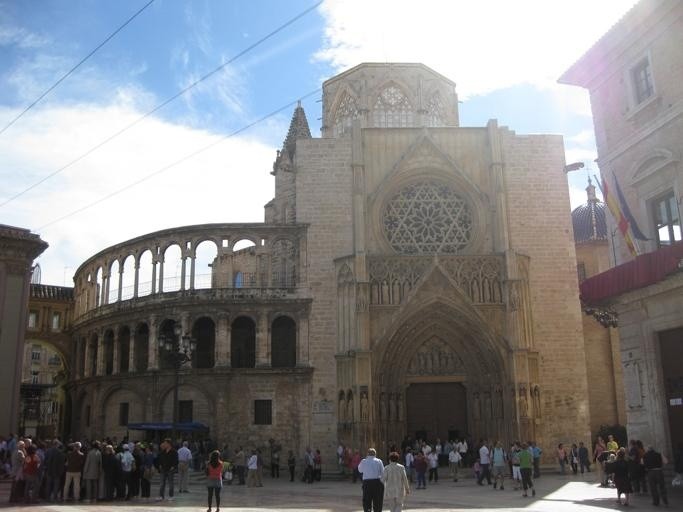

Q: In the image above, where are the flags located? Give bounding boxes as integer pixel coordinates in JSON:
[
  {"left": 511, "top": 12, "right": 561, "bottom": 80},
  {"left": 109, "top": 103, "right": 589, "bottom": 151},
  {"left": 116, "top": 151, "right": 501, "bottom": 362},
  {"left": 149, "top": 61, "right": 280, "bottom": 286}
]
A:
[{"left": 600, "top": 176, "right": 653, "bottom": 258}]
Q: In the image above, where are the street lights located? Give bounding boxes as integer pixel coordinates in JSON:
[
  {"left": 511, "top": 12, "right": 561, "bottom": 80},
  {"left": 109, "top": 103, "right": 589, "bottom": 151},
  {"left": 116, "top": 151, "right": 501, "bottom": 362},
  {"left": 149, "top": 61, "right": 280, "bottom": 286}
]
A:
[{"left": 156, "top": 323, "right": 200, "bottom": 448}]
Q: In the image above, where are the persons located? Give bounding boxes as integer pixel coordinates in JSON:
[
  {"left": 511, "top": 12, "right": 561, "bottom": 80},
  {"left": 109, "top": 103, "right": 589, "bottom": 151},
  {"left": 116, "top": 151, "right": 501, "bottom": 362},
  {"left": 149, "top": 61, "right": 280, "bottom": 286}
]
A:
[
  {"left": 386, "top": 433, "right": 468, "bottom": 489},
  {"left": 557, "top": 442, "right": 590, "bottom": 474},
  {"left": 358, "top": 448, "right": 410, "bottom": 511},
  {"left": 1, "top": 433, "right": 202, "bottom": 503},
  {"left": 206, "top": 450, "right": 224, "bottom": 511},
  {"left": 288, "top": 448, "right": 321, "bottom": 484},
  {"left": 474, "top": 438, "right": 543, "bottom": 498},
  {"left": 336, "top": 445, "right": 364, "bottom": 484},
  {"left": 221, "top": 439, "right": 281, "bottom": 489},
  {"left": 592, "top": 434, "right": 669, "bottom": 508}
]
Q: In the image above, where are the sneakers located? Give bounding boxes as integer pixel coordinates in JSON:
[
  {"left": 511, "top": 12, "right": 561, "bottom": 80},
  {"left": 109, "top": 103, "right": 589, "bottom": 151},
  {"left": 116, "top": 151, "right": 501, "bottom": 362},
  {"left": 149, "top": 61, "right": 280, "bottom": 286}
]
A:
[
  {"left": 616, "top": 500, "right": 629, "bottom": 506},
  {"left": 514, "top": 486, "right": 523, "bottom": 490},
  {"left": 166, "top": 497, "right": 175, "bottom": 502},
  {"left": 154, "top": 496, "right": 164, "bottom": 502},
  {"left": 415, "top": 486, "right": 426, "bottom": 489},
  {"left": 475, "top": 481, "right": 505, "bottom": 490},
  {"left": 522, "top": 494, "right": 528, "bottom": 497},
  {"left": 207, "top": 509, "right": 211, "bottom": 512},
  {"left": 215, "top": 509, "right": 219, "bottom": 512},
  {"left": 532, "top": 489, "right": 536, "bottom": 496},
  {"left": 179, "top": 488, "right": 190, "bottom": 493}
]
